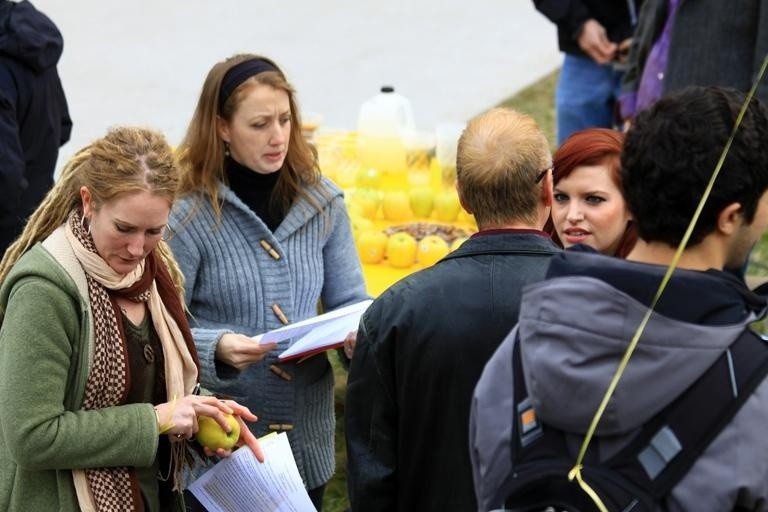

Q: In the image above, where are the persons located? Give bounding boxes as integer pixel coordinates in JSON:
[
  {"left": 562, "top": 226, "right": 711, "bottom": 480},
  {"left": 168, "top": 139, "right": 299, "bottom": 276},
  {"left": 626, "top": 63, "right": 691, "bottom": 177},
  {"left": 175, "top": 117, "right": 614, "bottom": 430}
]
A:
[
  {"left": 343, "top": 105, "right": 567, "bottom": 512},
  {"left": 530, "top": 0, "right": 644, "bottom": 146},
  {"left": 159, "top": 52, "right": 380, "bottom": 512},
  {"left": 541, "top": 125, "right": 639, "bottom": 259},
  {"left": 0, "top": 122, "right": 265, "bottom": 512},
  {"left": 2, "top": 0, "right": 73, "bottom": 265},
  {"left": 467, "top": 85, "right": 768, "bottom": 512},
  {"left": 615, "top": 0, "right": 765, "bottom": 135}
]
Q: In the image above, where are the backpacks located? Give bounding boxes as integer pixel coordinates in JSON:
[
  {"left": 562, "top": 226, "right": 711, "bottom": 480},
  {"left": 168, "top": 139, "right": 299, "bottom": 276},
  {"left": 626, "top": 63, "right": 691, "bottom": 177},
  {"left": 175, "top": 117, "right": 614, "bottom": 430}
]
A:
[{"left": 482, "top": 312, "right": 768, "bottom": 512}]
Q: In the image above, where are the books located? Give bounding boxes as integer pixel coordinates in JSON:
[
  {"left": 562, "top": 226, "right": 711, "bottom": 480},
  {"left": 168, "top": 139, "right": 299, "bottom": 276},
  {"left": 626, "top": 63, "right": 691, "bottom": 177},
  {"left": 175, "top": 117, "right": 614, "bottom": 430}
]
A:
[{"left": 252, "top": 299, "right": 375, "bottom": 361}]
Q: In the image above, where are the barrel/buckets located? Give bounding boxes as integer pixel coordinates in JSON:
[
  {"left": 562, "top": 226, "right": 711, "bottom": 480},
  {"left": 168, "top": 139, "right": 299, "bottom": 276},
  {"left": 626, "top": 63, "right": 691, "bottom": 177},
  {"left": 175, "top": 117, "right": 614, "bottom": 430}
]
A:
[{"left": 357, "top": 89, "right": 414, "bottom": 148}]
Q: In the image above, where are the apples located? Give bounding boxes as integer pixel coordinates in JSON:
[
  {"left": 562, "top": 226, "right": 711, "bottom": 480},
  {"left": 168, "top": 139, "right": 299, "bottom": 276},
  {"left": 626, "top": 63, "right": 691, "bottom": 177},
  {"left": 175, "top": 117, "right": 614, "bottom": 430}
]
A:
[
  {"left": 306, "top": 130, "right": 477, "bottom": 269},
  {"left": 193, "top": 411, "right": 240, "bottom": 454}
]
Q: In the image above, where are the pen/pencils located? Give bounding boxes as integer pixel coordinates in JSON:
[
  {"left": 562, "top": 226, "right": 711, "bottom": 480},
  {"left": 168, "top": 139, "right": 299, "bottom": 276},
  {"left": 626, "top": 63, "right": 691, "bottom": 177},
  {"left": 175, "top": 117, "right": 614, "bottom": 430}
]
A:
[{"left": 178, "top": 383, "right": 200, "bottom": 438}]
